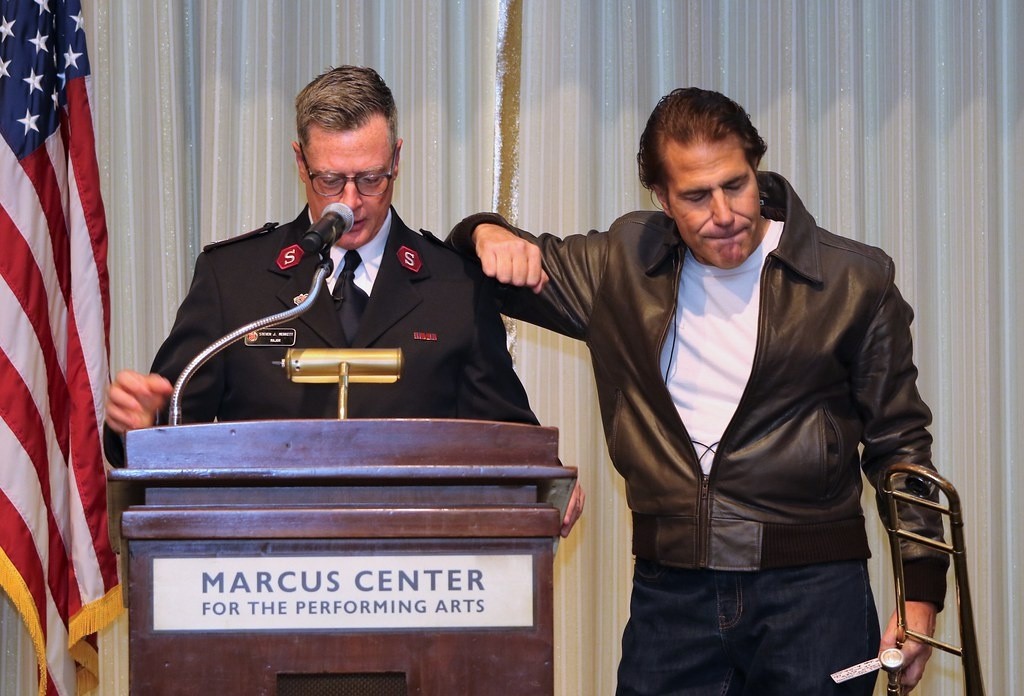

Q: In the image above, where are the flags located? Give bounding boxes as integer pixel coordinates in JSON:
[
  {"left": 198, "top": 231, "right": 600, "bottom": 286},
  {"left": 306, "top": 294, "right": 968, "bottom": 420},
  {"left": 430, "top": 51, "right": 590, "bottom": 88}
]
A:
[{"left": 0, "top": 0, "right": 122, "bottom": 696}]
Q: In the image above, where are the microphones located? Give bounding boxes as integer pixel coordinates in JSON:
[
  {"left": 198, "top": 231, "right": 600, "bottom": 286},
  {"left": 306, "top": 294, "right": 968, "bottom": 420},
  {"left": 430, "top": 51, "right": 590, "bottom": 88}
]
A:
[{"left": 304, "top": 203, "right": 354, "bottom": 255}]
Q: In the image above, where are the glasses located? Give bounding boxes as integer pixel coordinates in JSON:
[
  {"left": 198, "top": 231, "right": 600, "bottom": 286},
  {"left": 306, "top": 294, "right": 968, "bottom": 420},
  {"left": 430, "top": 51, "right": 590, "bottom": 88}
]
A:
[{"left": 299, "top": 142, "right": 397, "bottom": 197}]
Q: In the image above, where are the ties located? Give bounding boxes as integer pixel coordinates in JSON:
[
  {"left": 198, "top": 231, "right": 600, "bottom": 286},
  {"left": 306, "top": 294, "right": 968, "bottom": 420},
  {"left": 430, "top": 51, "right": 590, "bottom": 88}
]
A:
[{"left": 332, "top": 249, "right": 369, "bottom": 346}]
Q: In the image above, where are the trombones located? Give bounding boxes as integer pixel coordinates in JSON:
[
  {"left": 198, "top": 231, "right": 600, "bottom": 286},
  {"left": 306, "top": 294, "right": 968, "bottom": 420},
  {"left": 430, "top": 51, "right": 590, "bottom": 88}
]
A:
[{"left": 880, "top": 461, "right": 986, "bottom": 696}]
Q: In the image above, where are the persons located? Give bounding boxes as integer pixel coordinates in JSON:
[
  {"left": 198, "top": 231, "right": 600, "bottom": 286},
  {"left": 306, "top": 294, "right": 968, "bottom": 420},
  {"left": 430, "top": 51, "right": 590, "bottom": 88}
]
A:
[
  {"left": 105, "top": 65, "right": 585, "bottom": 539},
  {"left": 444, "top": 88, "right": 949, "bottom": 696}
]
